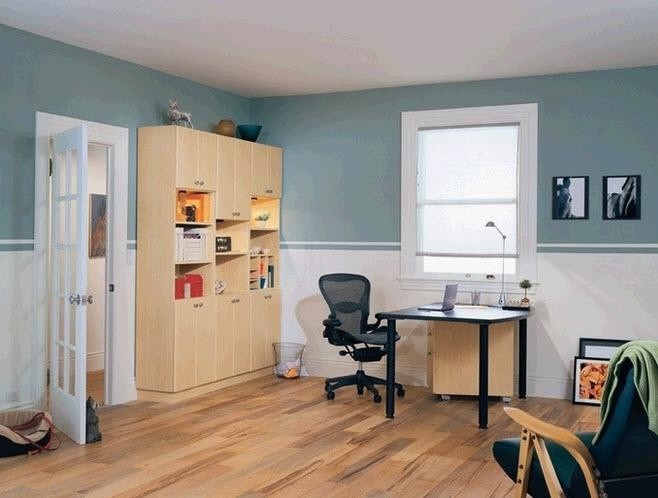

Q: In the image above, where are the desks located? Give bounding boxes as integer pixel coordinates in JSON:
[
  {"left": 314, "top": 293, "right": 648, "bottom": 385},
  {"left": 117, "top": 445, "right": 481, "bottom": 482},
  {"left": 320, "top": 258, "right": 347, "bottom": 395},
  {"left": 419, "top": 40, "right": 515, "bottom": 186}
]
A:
[{"left": 375, "top": 301, "right": 534, "bottom": 428}]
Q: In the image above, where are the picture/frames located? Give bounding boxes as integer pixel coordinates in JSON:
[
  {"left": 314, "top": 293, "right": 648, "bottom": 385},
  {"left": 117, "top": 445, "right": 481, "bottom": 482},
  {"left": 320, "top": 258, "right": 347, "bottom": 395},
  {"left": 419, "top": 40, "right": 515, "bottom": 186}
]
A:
[
  {"left": 551, "top": 175, "right": 589, "bottom": 220},
  {"left": 579, "top": 338, "right": 632, "bottom": 360},
  {"left": 572, "top": 355, "right": 612, "bottom": 406},
  {"left": 602, "top": 174, "right": 641, "bottom": 221},
  {"left": 89, "top": 193, "right": 107, "bottom": 259}
]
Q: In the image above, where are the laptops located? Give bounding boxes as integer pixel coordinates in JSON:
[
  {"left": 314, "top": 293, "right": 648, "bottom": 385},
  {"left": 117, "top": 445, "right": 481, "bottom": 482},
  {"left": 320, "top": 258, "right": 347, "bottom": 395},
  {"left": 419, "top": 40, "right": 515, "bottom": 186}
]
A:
[{"left": 418, "top": 284, "right": 457, "bottom": 311}]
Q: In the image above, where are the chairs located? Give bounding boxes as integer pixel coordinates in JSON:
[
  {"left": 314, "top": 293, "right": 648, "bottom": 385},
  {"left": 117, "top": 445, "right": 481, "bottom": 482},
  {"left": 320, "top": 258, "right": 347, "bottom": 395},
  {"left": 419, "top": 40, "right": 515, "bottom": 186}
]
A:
[
  {"left": 492, "top": 339, "right": 658, "bottom": 498},
  {"left": 319, "top": 272, "right": 406, "bottom": 402}
]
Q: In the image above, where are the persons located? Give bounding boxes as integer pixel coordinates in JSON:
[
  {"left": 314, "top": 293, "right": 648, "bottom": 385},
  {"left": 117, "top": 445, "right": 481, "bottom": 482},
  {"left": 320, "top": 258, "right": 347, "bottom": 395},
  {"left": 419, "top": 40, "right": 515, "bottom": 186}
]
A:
[{"left": 556, "top": 177, "right": 575, "bottom": 217}]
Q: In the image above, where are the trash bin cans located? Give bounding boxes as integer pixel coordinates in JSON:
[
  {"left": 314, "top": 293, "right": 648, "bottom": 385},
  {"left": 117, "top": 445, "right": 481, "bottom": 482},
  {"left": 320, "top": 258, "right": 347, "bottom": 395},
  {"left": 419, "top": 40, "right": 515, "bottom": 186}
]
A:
[{"left": 273, "top": 342, "right": 306, "bottom": 379}]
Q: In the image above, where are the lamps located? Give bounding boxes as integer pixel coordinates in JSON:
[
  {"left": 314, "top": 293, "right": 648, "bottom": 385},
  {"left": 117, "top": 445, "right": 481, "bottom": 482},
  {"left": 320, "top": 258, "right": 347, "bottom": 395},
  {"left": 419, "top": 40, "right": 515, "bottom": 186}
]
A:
[{"left": 483, "top": 220, "right": 511, "bottom": 307}]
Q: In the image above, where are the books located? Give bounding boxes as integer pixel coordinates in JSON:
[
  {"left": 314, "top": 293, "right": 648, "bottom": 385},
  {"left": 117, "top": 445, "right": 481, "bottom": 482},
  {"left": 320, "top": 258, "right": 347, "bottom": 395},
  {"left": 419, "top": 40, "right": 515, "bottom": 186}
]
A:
[
  {"left": 260, "top": 260, "right": 264, "bottom": 274},
  {"left": 268, "top": 264, "right": 274, "bottom": 287},
  {"left": 269, "top": 271, "right": 271, "bottom": 287},
  {"left": 260, "top": 277, "right": 267, "bottom": 288}
]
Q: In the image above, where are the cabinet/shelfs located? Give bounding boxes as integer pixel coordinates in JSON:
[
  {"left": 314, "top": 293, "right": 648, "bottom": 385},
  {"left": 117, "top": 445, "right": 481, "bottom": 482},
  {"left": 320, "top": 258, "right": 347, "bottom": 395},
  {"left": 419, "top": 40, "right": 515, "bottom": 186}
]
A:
[
  {"left": 216, "top": 134, "right": 250, "bottom": 380},
  {"left": 250, "top": 142, "right": 283, "bottom": 371},
  {"left": 137, "top": 125, "right": 216, "bottom": 394},
  {"left": 426, "top": 319, "right": 517, "bottom": 402}
]
